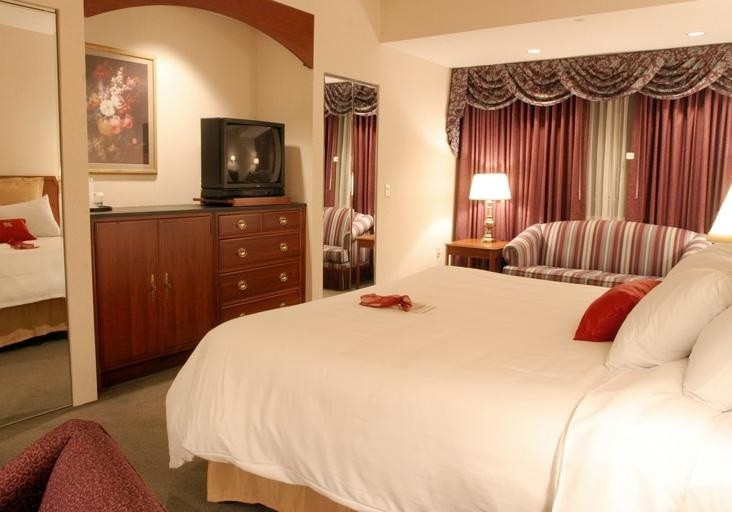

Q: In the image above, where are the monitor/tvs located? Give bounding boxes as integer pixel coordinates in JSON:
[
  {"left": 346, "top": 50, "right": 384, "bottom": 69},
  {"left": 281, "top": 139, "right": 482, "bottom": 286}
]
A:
[{"left": 200, "top": 117, "right": 286, "bottom": 199}]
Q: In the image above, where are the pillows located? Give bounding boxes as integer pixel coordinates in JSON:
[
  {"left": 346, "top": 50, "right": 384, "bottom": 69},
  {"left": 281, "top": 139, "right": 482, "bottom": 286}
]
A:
[
  {"left": 324, "top": 207, "right": 355, "bottom": 247},
  {"left": 602, "top": 243, "right": 732, "bottom": 378},
  {"left": 572, "top": 279, "right": 663, "bottom": 341},
  {"left": 682, "top": 306, "right": 732, "bottom": 414}
]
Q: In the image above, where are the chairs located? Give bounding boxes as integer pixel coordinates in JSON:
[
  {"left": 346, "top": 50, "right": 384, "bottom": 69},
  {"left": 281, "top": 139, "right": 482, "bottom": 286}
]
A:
[{"left": 0, "top": 418, "right": 168, "bottom": 512}]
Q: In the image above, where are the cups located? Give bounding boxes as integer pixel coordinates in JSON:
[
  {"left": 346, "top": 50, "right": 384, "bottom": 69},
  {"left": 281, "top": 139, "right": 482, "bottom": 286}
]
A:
[{"left": 93, "top": 192, "right": 103, "bottom": 206}]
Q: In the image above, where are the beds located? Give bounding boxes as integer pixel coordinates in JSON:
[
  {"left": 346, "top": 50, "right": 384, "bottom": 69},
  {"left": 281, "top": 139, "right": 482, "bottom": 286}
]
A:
[
  {"left": 0, "top": 174, "right": 69, "bottom": 348},
  {"left": 164, "top": 263, "right": 730, "bottom": 512}
]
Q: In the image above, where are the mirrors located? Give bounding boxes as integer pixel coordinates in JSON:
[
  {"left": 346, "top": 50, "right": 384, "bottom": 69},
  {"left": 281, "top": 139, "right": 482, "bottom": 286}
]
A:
[
  {"left": 324, "top": 73, "right": 380, "bottom": 292},
  {"left": 0, "top": 0, "right": 75, "bottom": 428}
]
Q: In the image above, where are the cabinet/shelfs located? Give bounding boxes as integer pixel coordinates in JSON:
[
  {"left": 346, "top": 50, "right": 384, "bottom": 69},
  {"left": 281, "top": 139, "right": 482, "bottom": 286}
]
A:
[
  {"left": 93, "top": 211, "right": 218, "bottom": 369},
  {"left": 214, "top": 205, "right": 308, "bottom": 325}
]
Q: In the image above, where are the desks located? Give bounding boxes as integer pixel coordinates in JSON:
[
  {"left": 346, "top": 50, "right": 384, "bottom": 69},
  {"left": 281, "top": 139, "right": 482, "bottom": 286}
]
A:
[{"left": 445, "top": 238, "right": 510, "bottom": 274}]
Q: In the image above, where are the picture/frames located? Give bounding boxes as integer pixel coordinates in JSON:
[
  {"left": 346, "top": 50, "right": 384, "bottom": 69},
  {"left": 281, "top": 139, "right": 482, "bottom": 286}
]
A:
[{"left": 83, "top": 40, "right": 159, "bottom": 175}]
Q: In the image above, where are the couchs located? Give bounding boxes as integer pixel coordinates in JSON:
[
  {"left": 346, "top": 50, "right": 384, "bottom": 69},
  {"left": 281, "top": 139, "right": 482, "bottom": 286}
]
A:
[
  {"left": 324, "top": 205, "right": 374, "bottom": 291},
  {"left": 500, "top": 220, "right": 713, "bottom": 290}
]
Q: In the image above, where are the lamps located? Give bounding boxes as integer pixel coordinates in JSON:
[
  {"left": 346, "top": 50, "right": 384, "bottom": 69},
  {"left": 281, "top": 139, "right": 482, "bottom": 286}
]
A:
[
  {"left": 705, "top": 184, "right": 732, "bottom": 242},
  {"left": 468, "top": 173, "right": 512, "bottom": 245}
]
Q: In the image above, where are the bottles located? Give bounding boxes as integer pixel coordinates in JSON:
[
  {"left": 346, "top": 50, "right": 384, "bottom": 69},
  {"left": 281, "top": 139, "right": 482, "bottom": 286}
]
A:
[{"left": 89, "top": 176, "right": 94, "bottom": 207}]
